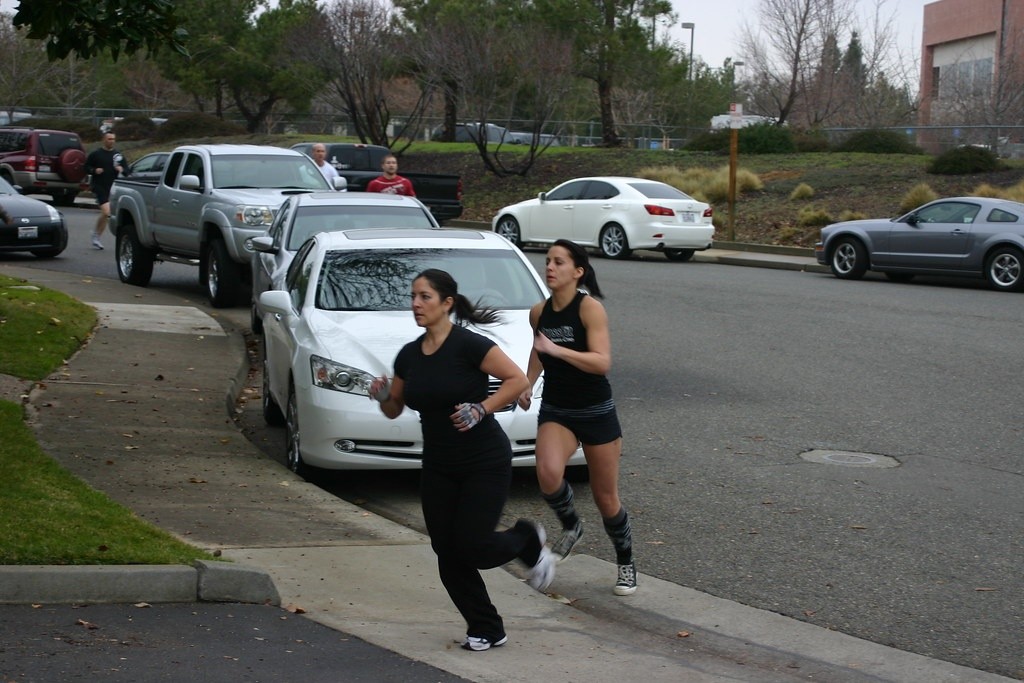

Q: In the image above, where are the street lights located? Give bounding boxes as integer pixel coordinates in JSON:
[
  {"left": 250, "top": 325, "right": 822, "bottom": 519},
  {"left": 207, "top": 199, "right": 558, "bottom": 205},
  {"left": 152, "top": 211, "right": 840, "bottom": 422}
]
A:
[
  {"left": 681, "top": 22, "right": 695, "bottom": 80},
  {"left": 733, "top": 61, "right": 744, "bottom": 70}
]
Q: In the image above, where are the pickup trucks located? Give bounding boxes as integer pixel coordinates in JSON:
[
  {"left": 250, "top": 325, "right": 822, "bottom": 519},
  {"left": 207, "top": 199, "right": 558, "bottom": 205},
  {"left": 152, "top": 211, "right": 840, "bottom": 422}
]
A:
[
  {"left": 288, "top": 141, "right": 463, "bottom": 226},
  {"left": 107, "top": 144, "right": 349, "bottom": 310}
]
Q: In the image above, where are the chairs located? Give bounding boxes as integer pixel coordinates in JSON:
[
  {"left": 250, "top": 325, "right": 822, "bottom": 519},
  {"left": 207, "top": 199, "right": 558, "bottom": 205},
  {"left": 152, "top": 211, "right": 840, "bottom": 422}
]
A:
[{"left": 445, "top": 259, "right": 511, "bottom": 306}]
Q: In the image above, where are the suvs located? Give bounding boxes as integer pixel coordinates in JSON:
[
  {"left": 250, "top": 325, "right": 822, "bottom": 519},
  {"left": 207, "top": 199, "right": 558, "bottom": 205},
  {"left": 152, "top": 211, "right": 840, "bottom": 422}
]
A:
[{"left": 0, "top": 124, "right": 93, "bottom": 207}]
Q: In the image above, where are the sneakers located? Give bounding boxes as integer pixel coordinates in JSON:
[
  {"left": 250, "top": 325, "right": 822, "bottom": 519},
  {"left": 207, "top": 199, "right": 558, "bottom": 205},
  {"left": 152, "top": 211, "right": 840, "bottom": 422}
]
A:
[
  {"left": 461, "top": 634, "right": 507, "bottom": 651},
  {"left": 551, "top": 520, "right": 585, "bottom": 565},
  {"left": 614, "top": 560, "right": 637, "bottom": 596},
  {"left": 89, "top": 231, "right": 104, "bottom": 250},
  {"left": 525, "top": 521, "right": 556, "bottom": 592}
]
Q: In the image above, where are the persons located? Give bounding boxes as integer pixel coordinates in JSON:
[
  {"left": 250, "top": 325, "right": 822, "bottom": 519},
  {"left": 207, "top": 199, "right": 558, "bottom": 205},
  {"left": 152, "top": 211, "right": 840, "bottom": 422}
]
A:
[
  {"left": 366, "top": 153, "right": 417, "bottom": 198},
  {"left": 368, "top": 269, "right": 531, "bottom": 652},
  {"left": 308, "top": 144, "right": 341, "bottom": 194},
  {"left": 82, "top": 131, "right": 132, "bottom": 250},
  {"left": 517, "top": 238, "right": 638, "bottom": 596}
]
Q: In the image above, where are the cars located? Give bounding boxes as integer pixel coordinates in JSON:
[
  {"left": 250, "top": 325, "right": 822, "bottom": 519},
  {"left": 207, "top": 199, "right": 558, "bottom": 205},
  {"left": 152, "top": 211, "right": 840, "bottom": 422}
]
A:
[
  {"left": 117, "top": 151, "right": 171, "bottom": 185},
  {"left": 0, "top": 175, "right": 69, "bottom": 261},
  {"left": 431, "top": 121, "right": 560, "bottom": 146},
  {"left": 259, "top": 227, "right": 594, "bottom": 484},
  {"left": 0, "top": 110, "right": 30, "bottom": 125},
  {"left": 492, "top": 176, "right": 715, "bottom": 260},
  {"left": 814, "top": 197, "right": 1024, "bottom": 294},
  {"left": 99, "top": 119, "right": 112, "bottom": 133},
  {"left": 250, "top": 192, "right": 440, "bottom": 337}
]
